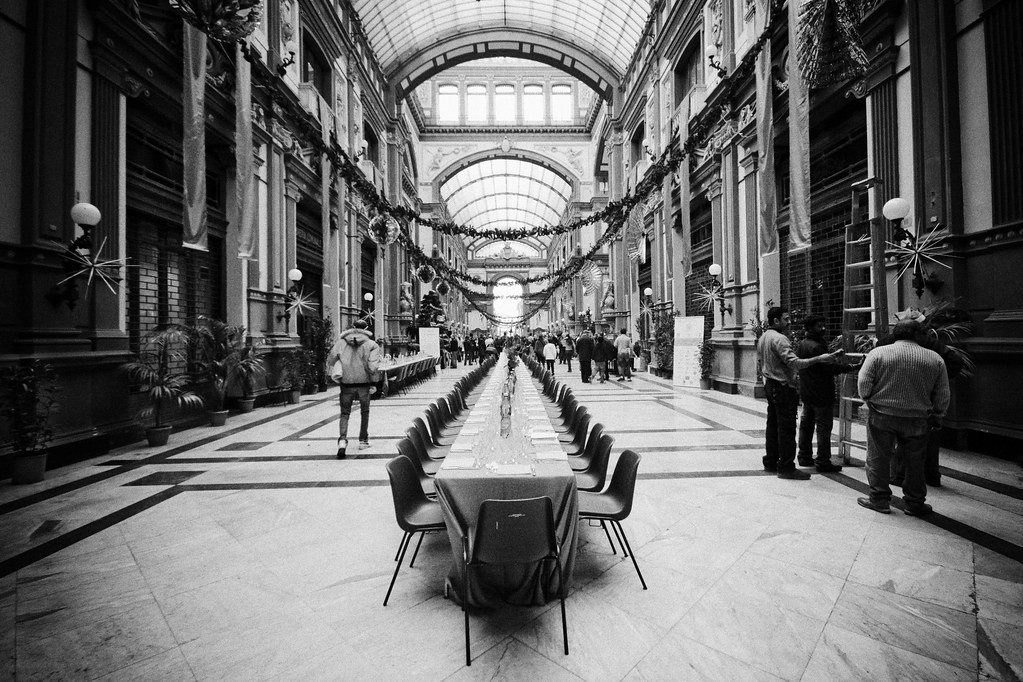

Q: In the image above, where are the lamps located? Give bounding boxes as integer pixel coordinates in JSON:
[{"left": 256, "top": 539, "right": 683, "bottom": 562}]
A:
[
  {"left": 287, "top": 268, "right": 303, "bottom": 297},
  {"left": 642, "top": 139, "right": 656, "bottom": 159},
  {"left": 709, "top": 264, "right": 722, "bottom": 283},
  {"left": 644, "top": 287, "right": 654, "bottom": 303},
  {"left": 706, "top": 44, "right": 725, "bottom": 77},
  {"left": 354, "top": 139, "right": 369, "bottom": 163},
  {"left": 277, "top": 41, "right": 296, "bottom": 76},
  {"left": 883, "top": 198, "right": 911, "bottom": 240},
  {"left": 72, "top": 202, "right": 101, "bottom": 252},
  {"left": 364, "top": 292, "right": 373, "bottom": 310}
]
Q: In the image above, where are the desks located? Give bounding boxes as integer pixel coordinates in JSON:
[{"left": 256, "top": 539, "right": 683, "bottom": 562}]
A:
[
  {"left": 434, "top": 350, "right": 580, "bottom": 602},
  {"left": 380, "top": 355, "right": 431, "bottom": 372}
]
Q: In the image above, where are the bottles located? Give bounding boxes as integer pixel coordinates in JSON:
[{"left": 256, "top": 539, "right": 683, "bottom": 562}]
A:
[{"left": 500, "top": 369, "right": 516, "bottom": 438}]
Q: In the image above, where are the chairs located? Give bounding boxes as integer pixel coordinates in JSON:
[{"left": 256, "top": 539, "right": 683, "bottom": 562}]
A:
[
  {"left": 521, "top": 351, "right": 647, "bottom": 590},
  {"left": 461, "top": 496, "right": 569, "bottom": 666},
  {"left": 383, "top": 354, "right": 499, "bottom": 603},
  {"left": 266, "top": 373, "right": 284, "bottom": 406}
]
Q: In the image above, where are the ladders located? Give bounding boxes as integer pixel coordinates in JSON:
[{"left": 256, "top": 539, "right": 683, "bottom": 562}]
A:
[{"left": 839, "top": 177, "right": 890, "bottom": 464}]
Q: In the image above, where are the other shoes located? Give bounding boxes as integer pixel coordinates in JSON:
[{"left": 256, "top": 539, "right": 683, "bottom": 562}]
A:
[
  {"left": 858, "top": 497, "right": 892, "bottom": 513},
  {"left": 798, "top": 458, "right": 816, "bottom": 467},
  {"left": 777, "top": 466, "right": 810, "bottom": 480},
  {"left": 358, "top": 439, "right": 371, "bottom": 450},
  {"left": 815, "top": 462, "right": 842, "bottom": 473},
  {"left": 925, "top": 474, "right": 941, "bottom": 487},
  {"left": 581, "top": 368, "right": 637, "bottom": 383},
  {"left": 336, "top": 439, "right": 349, "bottom": 458},
  {"left": 904, "top": 504, "right": 932, "bottom": 514},
  {"left": 889, "top": 474, "right": 905, "bottom": 487},
  {"left": 763, "top": 463, "right": 779, "bottom": 473}
]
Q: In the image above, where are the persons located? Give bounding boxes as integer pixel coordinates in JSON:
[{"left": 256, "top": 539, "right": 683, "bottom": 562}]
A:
[
  {"left": 327, "top": 320, "right": 382, "bottom": 458},
  {"left": 757, "top": 307, "right": 836, "bottom": 479},
  {"left": 527, "top": 328, "right": 640, "bottom": 383},
  {"left": 448, "top": 333, "right": 503, "bottom": 369},
  {"left": 855, "top": 318, "right": 950, "bottom": 516},
  {"left": 406, "top": 321, "right": 416, "bottom": 339},
  {"left": 796, "top": 315, "right": 865, "bottom": 473}
]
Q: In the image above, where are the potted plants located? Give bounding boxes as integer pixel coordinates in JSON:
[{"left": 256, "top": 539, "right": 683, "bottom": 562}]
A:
[
  {"left": 0, "top": 360, "right": 63, "bottom": 485},
  {"left": 117, "top": 323, "right": 204, "bottom": 447},
  {"left": 284, "top": 348, "right": 307, "bottom": 404},
  {"left": 187, "top": 317, "right": 263, "bottom": 425},
  {"left": 236, "top": 342, "right": 261, "bottom": 412},
  {"left": 655, "top": 311, "right": 678, "bottom": 379},
  {"left": 312, "top": 319, "right": 332, "bottom": 392},
  {"left": 696, "top": 342, "right": 715, "bottom": 390},
  {"left": 306, "top": 350, "right": 319, "bottom": 394}
]
